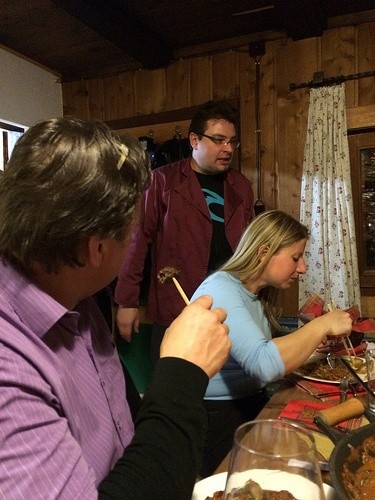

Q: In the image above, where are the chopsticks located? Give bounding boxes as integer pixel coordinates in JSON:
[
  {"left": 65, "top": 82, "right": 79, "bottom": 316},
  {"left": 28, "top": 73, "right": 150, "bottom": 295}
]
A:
[
  {"left": 326, "top": 300, "right": 357, "bottom": 362},
  {"left": 172, "top": 277, "right": 190, "bottom": 305}
]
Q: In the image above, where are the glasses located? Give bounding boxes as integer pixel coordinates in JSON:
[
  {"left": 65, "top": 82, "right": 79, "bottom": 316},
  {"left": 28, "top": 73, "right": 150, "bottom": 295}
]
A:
[
  {"left": 191, "top": 130, "right": 241, "bottom": 148},
  {"left": 115, "top": 134, "right": 131, "bottom": 169}
]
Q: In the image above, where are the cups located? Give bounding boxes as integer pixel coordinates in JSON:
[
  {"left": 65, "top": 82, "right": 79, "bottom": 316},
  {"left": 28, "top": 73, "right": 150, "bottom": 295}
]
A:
[{"left": 222, "top": 419, "right": 327, "bottom": 500}]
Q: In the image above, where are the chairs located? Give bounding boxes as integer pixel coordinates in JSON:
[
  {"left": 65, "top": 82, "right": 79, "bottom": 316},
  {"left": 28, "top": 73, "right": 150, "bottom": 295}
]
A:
[{"left": 114, "top": 322, "right": 167, "bottom": 419}]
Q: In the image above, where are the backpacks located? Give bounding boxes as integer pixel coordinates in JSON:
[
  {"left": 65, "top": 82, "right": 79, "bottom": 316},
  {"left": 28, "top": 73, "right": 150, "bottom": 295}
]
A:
[{"left": 150, "top": 134, "right": 190, "bottom": 170}]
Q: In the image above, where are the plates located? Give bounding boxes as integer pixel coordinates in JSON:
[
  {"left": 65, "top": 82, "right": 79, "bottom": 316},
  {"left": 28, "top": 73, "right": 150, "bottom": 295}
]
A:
[
  {"left": 291, "top": 353, "right": 375, "bottom": 383},
  {"left": 192, "top": 469, "right": 334, "bottom": 500}
]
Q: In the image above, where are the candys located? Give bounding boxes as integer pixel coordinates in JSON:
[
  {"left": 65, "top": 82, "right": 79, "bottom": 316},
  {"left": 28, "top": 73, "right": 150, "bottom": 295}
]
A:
[{"left": 158, "top": 266, "right": 191, "bottom": 307}]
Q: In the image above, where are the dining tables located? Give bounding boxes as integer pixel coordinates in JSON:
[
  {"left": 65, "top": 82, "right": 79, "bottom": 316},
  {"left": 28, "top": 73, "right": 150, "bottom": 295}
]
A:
[{"left": 210, "top": 373, "right": 375, "bottom": 489}]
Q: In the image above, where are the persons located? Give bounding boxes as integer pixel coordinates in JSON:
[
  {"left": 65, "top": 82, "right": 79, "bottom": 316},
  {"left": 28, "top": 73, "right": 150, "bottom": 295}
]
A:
[
  {"left": 184, "top": 209, "right": 356, "bottom": 479},
  {"left": 114, "top": 99, "right": 257, "bottom": 397},
  {"left": 0, "top": 117, "right": 235, "bottom": 500}
]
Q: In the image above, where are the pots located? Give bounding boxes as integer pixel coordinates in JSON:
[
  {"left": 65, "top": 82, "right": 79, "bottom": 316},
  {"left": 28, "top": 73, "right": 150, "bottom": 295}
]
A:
[{"left": 315, "top": 416, "right": 375, "bottom": 500}]
